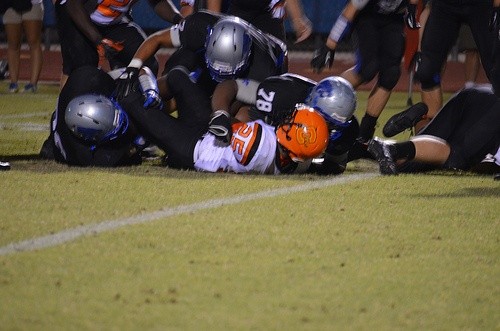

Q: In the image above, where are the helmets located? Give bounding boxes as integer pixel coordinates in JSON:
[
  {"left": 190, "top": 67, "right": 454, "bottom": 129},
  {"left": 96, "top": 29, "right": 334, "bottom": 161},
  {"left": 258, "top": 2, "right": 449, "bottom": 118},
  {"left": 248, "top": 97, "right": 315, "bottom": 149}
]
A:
[
  {"left": 64, "top": 94, "right": 128, "bottom": 141},
  {"left": 307, "top": 76, "right": 357, "bottom": 140},
  {"left": 276, "top": 104, "right": 329, "bottom": 163},
  {"left": 204, "top": 20, "right": 251, "bottom": 81}
]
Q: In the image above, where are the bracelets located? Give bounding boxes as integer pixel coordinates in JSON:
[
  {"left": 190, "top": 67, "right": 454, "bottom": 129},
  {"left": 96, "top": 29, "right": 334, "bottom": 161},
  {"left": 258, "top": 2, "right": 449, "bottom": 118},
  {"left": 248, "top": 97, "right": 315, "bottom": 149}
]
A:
[{"left": 128, "top": 58, "right": 142, "bottom": 68}]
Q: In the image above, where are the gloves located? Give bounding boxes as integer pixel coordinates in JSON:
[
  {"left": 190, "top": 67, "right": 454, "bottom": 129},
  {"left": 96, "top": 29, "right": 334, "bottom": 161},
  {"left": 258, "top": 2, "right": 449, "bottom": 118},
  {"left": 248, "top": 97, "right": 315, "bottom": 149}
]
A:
[
  {"left": 111, "top": 67, "right": 142, "bottom": 97},
  {"left": 409, "top": 51, "right": 421, "bottom": 83},
  {"left": 404, "top": 3, "right": 421, "bottom": 29},
  {"left": 310, "top": 45, "right": 335, "bottom": 71},
  {"left": 211, "top": 114, "right": 232, "bottom": 146},
  {"left": 142, "top": 89, "right": 163, "bottom": 110}
]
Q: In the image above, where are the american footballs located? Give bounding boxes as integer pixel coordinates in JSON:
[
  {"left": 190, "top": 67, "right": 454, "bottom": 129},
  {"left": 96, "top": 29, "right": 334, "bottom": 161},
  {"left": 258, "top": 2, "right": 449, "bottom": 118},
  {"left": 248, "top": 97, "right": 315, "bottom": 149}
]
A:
[{"left": 234, "top": 105, "right": 261, "bottom": 123}]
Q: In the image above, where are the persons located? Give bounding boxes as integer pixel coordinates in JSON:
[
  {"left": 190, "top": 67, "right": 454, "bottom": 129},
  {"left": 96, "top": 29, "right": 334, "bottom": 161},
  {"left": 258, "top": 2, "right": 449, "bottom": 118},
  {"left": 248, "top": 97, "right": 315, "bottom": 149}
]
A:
[
  {"left": 310, "top": 0, "right": 500, "bottom": 181},
  {"left": 3, "top": 0, "right": 44, "bottom": 93},
  {"left": 41, "top": 0, "right": 359, "bottom": 176}
]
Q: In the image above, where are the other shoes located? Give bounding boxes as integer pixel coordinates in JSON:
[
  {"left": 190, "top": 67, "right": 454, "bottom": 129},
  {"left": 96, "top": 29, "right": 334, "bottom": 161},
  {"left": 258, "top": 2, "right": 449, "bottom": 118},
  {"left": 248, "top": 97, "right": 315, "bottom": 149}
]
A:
[
  {"left": 9, "top": 82, "right": 18, "bottom": 92},
  {"left": 382, "top": 102, "right": 428, "bottom": 136},
  {"left": 369, "top": 137, "right": 395, "bottom": 174},
  {"left": 20, "top": 84, "right": 36, "bottom": 93}
]
